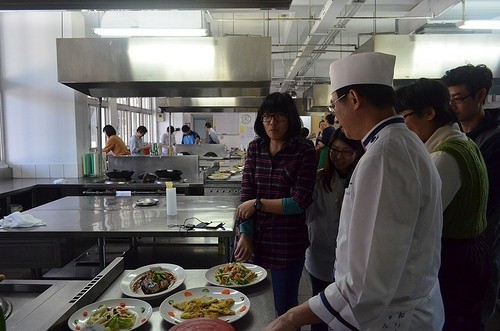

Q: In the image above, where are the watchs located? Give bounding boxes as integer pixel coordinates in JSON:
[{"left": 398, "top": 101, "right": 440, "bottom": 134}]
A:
[{"left": 253, "top": 198, "right": 262, "bottom": 211}]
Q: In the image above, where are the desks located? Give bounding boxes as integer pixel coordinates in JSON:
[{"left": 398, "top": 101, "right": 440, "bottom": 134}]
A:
[{"left": 0, "top": 195, "right": 242, "bottom": 280}]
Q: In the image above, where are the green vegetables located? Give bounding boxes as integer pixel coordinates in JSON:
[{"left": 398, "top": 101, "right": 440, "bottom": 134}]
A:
[
  {"left": 214, "top": 262, "right": 258, "bottom": 285},
  {"left": 151, "top": 271, "right": 165, "bottom": 281}
]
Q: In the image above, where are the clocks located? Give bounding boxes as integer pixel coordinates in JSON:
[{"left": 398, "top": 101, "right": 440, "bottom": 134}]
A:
[{"left": 240, "top": 114, "right": 251, "bottom": 124}]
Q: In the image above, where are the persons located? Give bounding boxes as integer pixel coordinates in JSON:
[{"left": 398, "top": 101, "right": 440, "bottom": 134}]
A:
[
  {"left": 442, "top": 64, "right": 500, "bottom": 331},
  {"left": 301, "top": 113, "right": 366, "bottom": 331},
  {"left": 262, "top": 52, "right": 445, "bottom": 331},
  {"left": 203, "top": 122, "right": 220, "bottom": 157},
  {"left": 102, "top": 125, "right": 131, "bottom": 156},
  {"left": 182, "top": 125, "right": 200, "bottom": 156},
  {"left": 394, "top": 78, "right": 488, "bottom": 331},
  {"left": 231, "top": 92, "right": 318, "bottom": 331},
  {"left": 130, "top": 125, "right": 152, "bottom": 155},
  {"left": 160, "top": 126, "right": 176, "bottom": 156}
]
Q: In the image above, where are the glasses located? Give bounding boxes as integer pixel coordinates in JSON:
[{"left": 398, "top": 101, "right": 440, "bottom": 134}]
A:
[
  {"left": 327, "top": 147, "right": 356, "bottom": 157},
  {"left": 448, "top": 94, "right": 472, "bottom": 105},
  {"left": 327, "top": 91, "right": 353, "bottom": 116},
  {"left": 260, "top": 113, "right": 289, "bottom": 123}
]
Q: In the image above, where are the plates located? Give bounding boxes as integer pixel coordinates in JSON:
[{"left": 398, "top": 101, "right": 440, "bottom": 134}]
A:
[
  {"left": 136, "top": 198, "right": 159, "bottom": 205},
  {"left": 120, "top": 263, "right": 185, "bottom": 297},
  {"left": 159, "top": 286, "right": 251, "bottom": 324},
  {"left": 68, "top": 298, "right": 153, "bottom": 331},
  {"left": 207, "top": 167, "right": 244, "bottom": 179},
  {"left": 205, "top": 262, "right": 267, "bottom": 287}
]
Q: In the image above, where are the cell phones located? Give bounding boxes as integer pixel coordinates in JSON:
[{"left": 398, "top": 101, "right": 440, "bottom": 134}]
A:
[{"left": 205, "top": 222, "right": 222, "bottom": 229}]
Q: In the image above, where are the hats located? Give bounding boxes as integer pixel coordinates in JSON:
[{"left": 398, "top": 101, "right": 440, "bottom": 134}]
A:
[{"left": 329, "top": 52, "right": 396, "bottom": 95}]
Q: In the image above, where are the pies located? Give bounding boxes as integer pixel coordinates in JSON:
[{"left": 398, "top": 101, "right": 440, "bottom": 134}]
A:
[{"left": 173, "top": 296, "right": 235, "bottom": 319}]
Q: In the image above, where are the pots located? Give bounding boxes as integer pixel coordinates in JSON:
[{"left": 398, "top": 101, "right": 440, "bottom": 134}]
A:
[
  {"left": 106, "top": 169, "right": 135, "bottom": 178},
  {"left": 155, "top": 169, "right": 183, "bottom": 179}
]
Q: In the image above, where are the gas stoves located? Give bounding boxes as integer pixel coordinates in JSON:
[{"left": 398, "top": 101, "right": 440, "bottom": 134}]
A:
[{"left": 97, "top": 178, "right": 189, "bottom": 189}]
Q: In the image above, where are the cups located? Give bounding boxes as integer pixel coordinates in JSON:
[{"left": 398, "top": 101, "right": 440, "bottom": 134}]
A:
[{"left": 166, "top": 188, "right": 177, "bottom": 215}]
